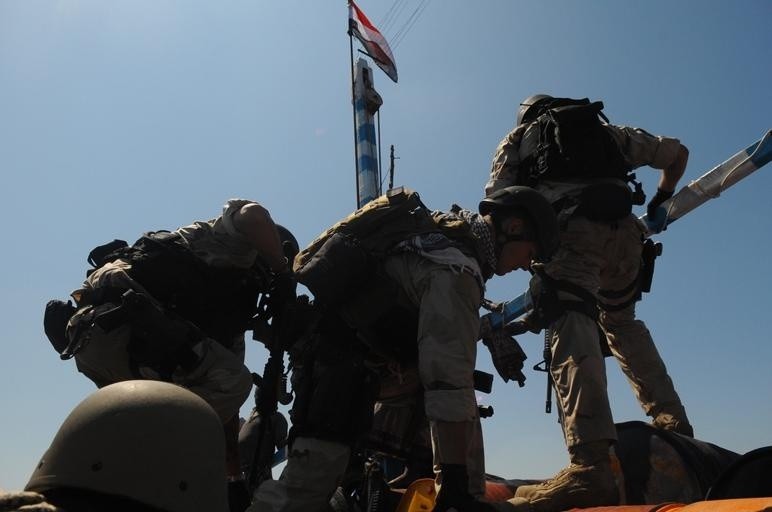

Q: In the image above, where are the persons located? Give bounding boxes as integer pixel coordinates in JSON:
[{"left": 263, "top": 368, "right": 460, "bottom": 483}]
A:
[
  {"left": 61, "top": 199, "right": 302, "bottom": 512},
  {"left": 239, "top": 387, "right": 289, "bottom": 496},
  {"left": 246, "top": 185, "right": 558, "bottom": 512},
  {"left": 484, "top": 96, "right": 694, "bottom": 505}
]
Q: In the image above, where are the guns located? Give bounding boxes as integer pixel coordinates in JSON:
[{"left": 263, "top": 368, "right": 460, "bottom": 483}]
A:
[{"left": 250, "top": 297, "right": 292, "bottom": 492}]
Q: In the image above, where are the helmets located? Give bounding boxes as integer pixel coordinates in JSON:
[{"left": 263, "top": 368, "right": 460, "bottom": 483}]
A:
[
  {"left": 516, "top": 95, "right": 553, "bottom": 126},
  {"left": 478, "top": 184, "right": 563, "bottom": 263}
]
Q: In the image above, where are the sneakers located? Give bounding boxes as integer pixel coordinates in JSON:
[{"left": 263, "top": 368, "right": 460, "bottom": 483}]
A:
[{"left": 510, "top": 457, "right": 621, "bottom": 509}]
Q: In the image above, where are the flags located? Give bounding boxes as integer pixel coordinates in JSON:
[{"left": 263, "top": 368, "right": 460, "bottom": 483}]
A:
[{"left": 351, "top": 1, "right": 399, "bottom": 83}]
